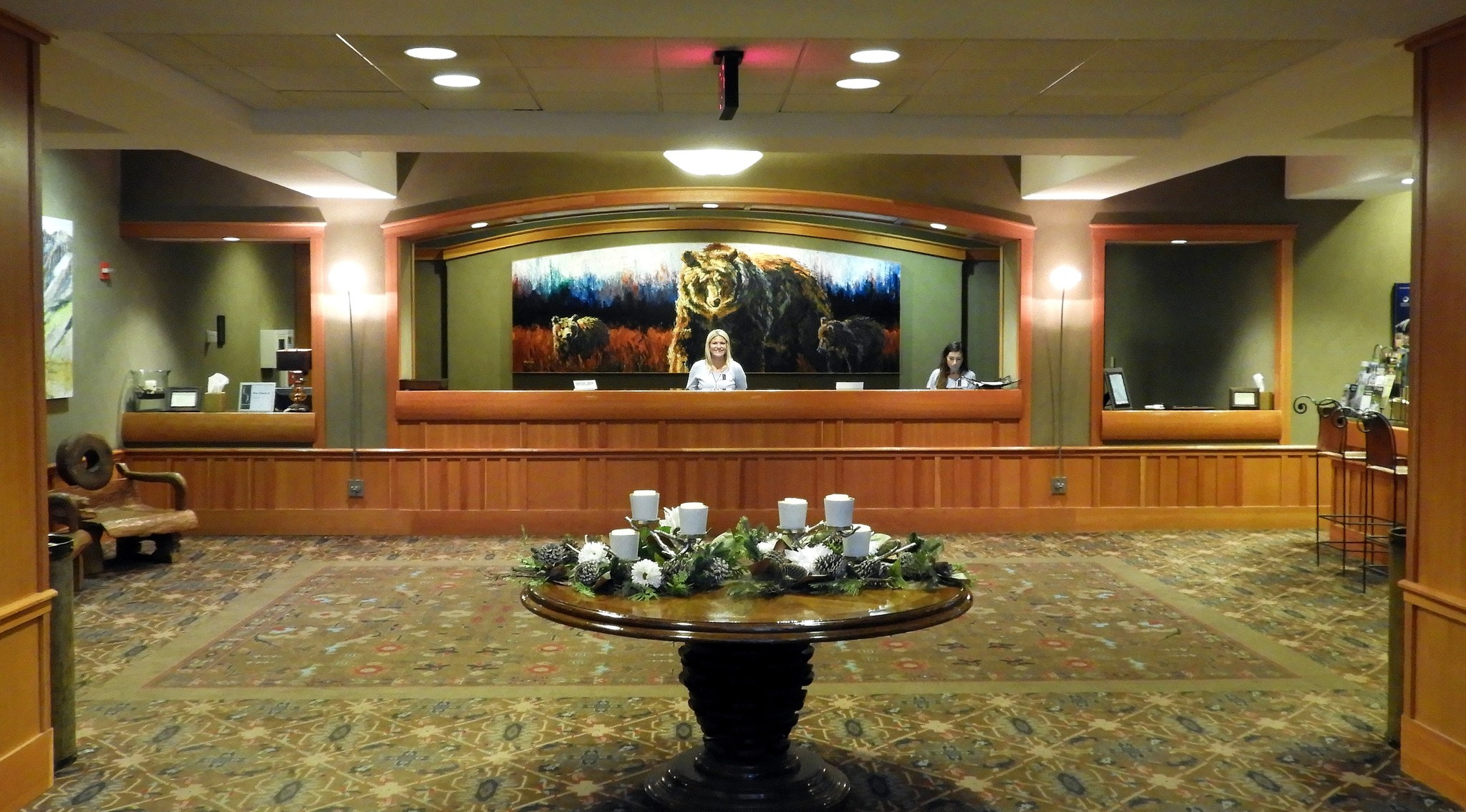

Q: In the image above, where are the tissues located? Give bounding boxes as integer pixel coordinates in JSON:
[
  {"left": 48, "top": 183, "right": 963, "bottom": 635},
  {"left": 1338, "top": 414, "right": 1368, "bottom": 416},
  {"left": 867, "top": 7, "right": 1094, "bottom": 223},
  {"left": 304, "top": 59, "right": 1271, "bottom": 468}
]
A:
[
  {"left": 204, "top": 372, "right": 229, "bottom": 412},
  {"left": 1252, "top": 373, "right": 1273, "bottom": 410}
]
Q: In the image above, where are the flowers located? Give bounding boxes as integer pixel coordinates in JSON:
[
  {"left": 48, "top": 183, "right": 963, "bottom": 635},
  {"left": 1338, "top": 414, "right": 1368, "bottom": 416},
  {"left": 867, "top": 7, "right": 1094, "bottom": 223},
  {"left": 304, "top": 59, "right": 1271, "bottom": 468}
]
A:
[{"left": 517, "top": 524, "right": 978, "bottom": 600}]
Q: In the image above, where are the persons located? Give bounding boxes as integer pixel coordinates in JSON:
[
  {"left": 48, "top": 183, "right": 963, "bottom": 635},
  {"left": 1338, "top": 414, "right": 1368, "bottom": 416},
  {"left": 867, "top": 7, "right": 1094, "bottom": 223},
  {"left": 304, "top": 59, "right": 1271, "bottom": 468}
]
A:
[
  {"left": 926, "top": 341, "right": 977, "bottom": 390},
  {"left": 686, "top": 328, "right": 747, "bottom": 392}
]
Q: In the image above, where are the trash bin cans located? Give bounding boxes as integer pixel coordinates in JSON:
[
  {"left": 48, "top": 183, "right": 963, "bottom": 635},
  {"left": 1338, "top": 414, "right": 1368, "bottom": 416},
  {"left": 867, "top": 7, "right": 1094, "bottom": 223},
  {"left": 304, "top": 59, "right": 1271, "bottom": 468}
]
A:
[
  {"left": 1387, "top": 527, "right": 1406, "bottom": 748},
  {"left": 48, "top": 533, "right": 77, "bottom": 772}
]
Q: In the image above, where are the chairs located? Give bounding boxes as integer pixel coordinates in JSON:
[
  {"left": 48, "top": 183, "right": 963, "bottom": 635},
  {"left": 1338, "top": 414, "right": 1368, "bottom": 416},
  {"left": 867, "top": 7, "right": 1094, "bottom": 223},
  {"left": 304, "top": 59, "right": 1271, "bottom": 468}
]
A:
[
  {"left": 47, "top": 460, "right": 198, "bottom": 568},
  {"left": 47, "top": 492, "right": 92, "bottom": 593}
]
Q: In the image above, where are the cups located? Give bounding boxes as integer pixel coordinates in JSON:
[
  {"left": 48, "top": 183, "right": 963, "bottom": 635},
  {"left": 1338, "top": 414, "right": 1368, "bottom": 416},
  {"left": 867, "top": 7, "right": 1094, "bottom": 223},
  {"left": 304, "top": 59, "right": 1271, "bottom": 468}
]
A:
[
  {"left": 608, "top": 530, "right": 640, "bottom": 561},
  {"left": 678, "top": 505, "right": 709, "bottom": 534},
  {"left": 842, "top": 530, "right": 871, "bottom": 557},
  {"left": 824, "top": 497, "right": 855, "bottom": 527},
  {"left": 629, "top": 492, "right": 660, "bottom": 520},
  {"left": 778, "top": 501, "right": 808, "bottom": 529}
]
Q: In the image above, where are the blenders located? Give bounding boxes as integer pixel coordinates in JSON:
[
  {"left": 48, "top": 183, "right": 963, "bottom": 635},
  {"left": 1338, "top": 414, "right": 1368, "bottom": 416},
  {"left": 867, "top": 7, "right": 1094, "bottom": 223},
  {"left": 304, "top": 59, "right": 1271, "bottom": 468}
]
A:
[{"left": 129, "top": 369, "right": 172, "bottom": 412}]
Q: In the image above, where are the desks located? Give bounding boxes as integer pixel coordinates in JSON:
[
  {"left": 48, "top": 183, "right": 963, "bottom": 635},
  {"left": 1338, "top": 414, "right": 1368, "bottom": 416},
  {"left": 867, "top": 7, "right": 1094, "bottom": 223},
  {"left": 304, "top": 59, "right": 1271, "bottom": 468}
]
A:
[{"left": 521, "top": 559, "right": 973, "bottom": 812}]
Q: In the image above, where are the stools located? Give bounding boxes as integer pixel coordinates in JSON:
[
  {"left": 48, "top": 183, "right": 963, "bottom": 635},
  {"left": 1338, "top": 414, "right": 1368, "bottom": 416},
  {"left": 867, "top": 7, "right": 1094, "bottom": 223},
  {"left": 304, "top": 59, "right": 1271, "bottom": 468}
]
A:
[{"left": 1294, "top": 395, "right": 1407, "bottom": 593}]
